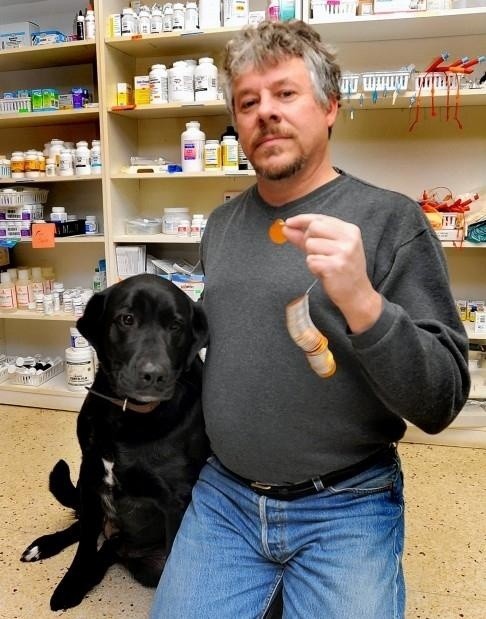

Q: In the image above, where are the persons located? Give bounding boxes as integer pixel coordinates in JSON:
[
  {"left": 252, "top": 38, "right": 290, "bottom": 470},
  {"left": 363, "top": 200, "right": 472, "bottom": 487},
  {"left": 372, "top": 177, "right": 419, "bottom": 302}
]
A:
[{"left": 145, "top": 15, "right": 474, "bottom": 618}]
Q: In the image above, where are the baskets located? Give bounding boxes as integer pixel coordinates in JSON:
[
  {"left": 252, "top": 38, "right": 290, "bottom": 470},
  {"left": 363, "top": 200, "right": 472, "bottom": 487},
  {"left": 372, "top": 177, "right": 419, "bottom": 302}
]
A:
[
  {"left": 341, "top": 71, "right": 459, "bottom": 93},
  {"left": 0, "top": 186, "right": 49, "bottom": 206},
  {"left": 0, "top": 97, "right": 32, "bottom": 114},
  {"left": 311, "top": 1, "right": 357, "bottom": 19},
  {"left": 442, "top": 212, "right": 459, "bottom": 229},
  {"left": 0, "top": 356, "right": 64, "bottom": 386},
  {"left": 0, "top": 165, "right": 11, "bottom": 177}
]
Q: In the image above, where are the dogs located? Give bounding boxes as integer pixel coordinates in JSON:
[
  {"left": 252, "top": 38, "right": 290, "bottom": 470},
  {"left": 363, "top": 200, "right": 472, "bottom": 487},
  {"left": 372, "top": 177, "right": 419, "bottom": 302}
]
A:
[{"left": 18, "top": 274, "right": 209, "bottom": 614}]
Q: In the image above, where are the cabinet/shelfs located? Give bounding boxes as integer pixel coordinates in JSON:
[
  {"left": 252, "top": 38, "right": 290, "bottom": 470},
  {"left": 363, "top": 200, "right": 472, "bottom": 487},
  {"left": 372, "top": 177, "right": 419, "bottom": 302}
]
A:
[{"left": 0, "top": 0, "right": 486, "bottom": 449}]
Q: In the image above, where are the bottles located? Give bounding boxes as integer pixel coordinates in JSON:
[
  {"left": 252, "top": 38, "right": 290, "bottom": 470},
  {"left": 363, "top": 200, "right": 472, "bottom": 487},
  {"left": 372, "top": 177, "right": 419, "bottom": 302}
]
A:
[
  {"left": 0, "top": 267, "right": 85, "bottom": 318},
  {"left": 5, "top": 189, "right": 99, "bottom": 239},
  {"left": 9, "top": 135, "right": 102, "bottom": 184},
  {"left": 75, "top": 8, "right": 95, "bottom": 43},
  {"left": 156, "top": 201, "right": 208, "bottom": 239},
  {"left": 182, "top": 122, "right": 244, "bottom": 175},
  {"left": 108, "top": 0, "right": 222, "bottom": 40},
  {"left": 152, "top": 57, "right": 221, "bottom": 112}
]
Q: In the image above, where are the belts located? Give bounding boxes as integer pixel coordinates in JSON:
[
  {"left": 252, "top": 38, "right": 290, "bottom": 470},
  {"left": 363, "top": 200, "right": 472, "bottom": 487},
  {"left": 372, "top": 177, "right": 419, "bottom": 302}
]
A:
[{"left": 224, "top": 447, "right": 390, "bottom": 501}]
